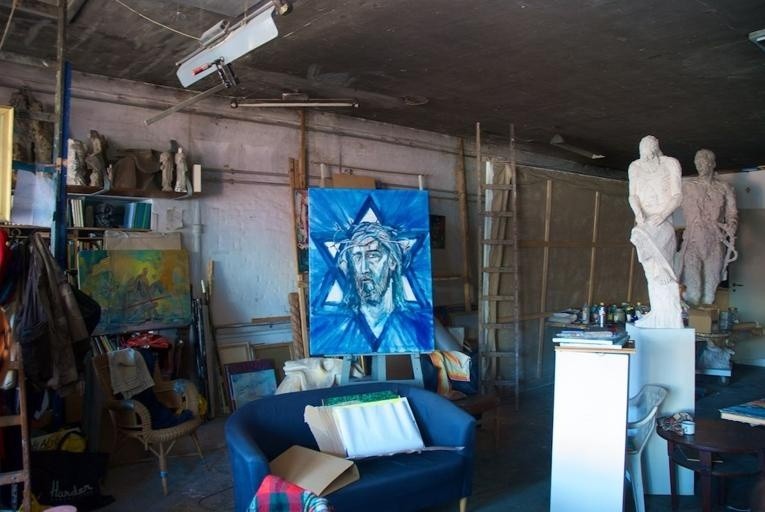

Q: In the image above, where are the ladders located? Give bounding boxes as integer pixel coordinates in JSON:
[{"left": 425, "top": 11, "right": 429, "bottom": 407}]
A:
[{"left": 476, "top": 122, "right": 521, "bottom": 412}]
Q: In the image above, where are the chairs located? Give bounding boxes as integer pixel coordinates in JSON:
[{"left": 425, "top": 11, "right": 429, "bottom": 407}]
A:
[
  {"left": 623, "top": 385, "right": 671, "bottom": 511},
  {"left": 93, "top": 350, "right": 209, "bottom": 498}
]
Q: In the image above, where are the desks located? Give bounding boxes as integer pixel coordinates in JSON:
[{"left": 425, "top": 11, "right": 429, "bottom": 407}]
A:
[
  {"left": 657, "top": 413, "right": 764, "bottom": 510},
  {"left": 685, "top": 285, "right": 761, "bottom": 385}
]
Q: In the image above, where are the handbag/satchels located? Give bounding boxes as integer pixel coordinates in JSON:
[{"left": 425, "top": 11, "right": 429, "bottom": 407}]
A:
[{"left": 29, "top": 430, "right": 114, "bottom": 512}]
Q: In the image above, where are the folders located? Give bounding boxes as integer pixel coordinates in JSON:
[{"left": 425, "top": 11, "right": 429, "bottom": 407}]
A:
[{"left": 270, "top": 445, "right": 361, "bottom": 497}]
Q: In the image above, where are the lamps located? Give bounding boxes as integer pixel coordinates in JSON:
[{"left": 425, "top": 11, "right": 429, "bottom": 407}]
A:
[{"left": 224, "top": 90, "right": 361, "bottom": 112}]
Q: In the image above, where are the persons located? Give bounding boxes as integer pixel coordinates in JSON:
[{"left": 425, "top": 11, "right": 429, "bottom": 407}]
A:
[
  {"left": 87, "top": 130, "right": 105, "bottom": 186},
  {"left": 627, "top": 135, "right": 684, "bottom": 329},
  {"left": 680, "top": 149, "right": 738, "bottom": 308},
  {"left": 174, "top": 146, "right": 189, "bottom": 192},
  {"left": 332, "top": 222, "right": 409, "bottom": 314}
]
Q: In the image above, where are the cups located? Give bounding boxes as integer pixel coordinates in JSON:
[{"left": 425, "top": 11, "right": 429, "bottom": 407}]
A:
[{"left": 682, "top": 421, "right": 697, "bottom": 437}]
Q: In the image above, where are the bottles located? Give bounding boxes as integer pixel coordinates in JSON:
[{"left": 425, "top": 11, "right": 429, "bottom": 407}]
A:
[{"left": 581, "top": 301, "right": 643, "bottom": 328}]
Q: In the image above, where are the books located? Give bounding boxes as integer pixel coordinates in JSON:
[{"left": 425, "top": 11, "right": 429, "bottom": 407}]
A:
[
  {"left": 553, "top": 330, "right": 631, "bottom": 350},
  {"left": 67, "top": 198, "right": 86, "bottom": 229},
  {"left": 719, "top": 398, "right": 765, "bottom": 426}
]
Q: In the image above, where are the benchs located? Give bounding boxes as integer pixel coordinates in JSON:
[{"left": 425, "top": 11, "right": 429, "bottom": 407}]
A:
[{"left": 223, "top": 383, "right": 482, "bottom": 512}]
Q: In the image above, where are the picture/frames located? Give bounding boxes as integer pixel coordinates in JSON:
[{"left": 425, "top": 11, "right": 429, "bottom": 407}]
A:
[{"left": 224, "top": 360, "right": 280, "bottom": 412}]
